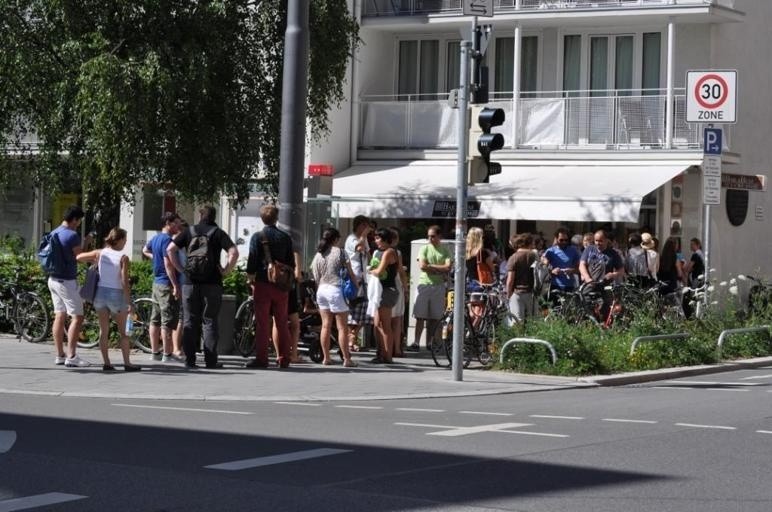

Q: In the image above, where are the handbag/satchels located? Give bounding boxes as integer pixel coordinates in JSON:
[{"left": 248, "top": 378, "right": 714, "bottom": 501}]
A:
[
  {"left": 267, "top": 260, "right": 296, "bottom": 288},
  {"left": 340, "top": 267, "right": 358, "bottom": 301},
  {"left": 476, "top": 259, "right": 495, "bottom": 284},
  {"left": 79, "top": 267, "right": 98, "bottom": 301}
]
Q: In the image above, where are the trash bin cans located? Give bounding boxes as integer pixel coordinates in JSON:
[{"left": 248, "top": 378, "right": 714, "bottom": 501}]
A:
[{"left": 200, "top": 295, "right": 237, "bottom": 354}]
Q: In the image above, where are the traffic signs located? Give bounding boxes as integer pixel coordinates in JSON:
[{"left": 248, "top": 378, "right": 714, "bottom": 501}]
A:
[{"left": 684, "top": 67, "right": 740, "bottom": 125}]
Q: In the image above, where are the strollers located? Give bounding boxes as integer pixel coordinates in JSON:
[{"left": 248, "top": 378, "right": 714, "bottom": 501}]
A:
[{"left": 295, "top": 280, "right": 370, "bottom": 364}]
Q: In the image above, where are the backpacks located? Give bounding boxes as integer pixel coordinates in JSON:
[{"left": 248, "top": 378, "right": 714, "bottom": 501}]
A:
[
  {"left": 38, "top": 227, "right": 69, "bottom": 276},
  {"left": 184, "top": 224, "right": 218, "bottom": 283}
]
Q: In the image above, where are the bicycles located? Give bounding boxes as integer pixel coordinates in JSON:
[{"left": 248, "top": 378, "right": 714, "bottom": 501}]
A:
[
  {"left": 1, "top": 264, "right": 48, "bottom": 346},
  {"left": 234, "top": 264, "right": 258, "bottom": 357},
  {"left": 61, "top": 275, "right": 164, "bottom": 354}
]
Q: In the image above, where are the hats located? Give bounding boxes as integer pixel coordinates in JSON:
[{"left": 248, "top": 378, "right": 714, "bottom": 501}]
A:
[{"left": 641, "top": 233, "right": 655, "bottom": 249}]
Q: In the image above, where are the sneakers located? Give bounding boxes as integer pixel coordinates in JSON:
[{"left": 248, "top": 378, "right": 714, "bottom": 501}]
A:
[
  {"left": 343, "top": 361, "right": 357, "bottom": 367},
  {"left": 63, "top": 355, "right": 91, "bottom": 367},
  {"left": 102, "top": 365, "right": 115, "bottom": 371},
  {"left": 246, "top": 357, "right": 303, "bottom": 370},
  {"left": 55, "top": 356, "right": 66, "bottom": 365},
  {"left": 151, "top": 351, "right": 223, "bottom": 369},
  {"left": 407, "top": 343, "right": 420, "bottom": 350},
  {"left": 372, "top": 346, "right": 406, "bottom": 364},
  {"left": 323, "top": 360, "right": 336, "bottom": 365},
  {"left": 124, "top": 364, "right": 141, "bottom": 371}
]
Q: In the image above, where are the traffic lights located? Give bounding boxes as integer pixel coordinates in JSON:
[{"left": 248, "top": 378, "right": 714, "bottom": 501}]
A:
[{"left": 473, "top": 104, "right": 507, "bottom": 183}]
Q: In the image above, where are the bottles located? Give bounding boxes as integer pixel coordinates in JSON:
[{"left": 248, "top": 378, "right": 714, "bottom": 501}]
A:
[{"left": 125, "top": 312, "right": 134, "bottom": 336}]
